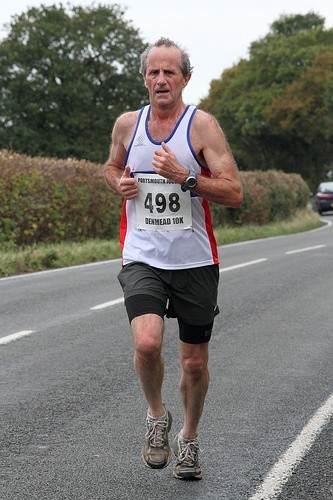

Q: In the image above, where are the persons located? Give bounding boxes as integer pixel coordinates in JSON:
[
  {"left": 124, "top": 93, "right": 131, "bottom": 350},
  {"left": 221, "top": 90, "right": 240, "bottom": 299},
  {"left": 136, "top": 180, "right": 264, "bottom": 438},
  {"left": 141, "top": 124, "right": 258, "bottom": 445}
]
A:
[{"left": 103, "top": 38, "right": 243, "bottom": 480}]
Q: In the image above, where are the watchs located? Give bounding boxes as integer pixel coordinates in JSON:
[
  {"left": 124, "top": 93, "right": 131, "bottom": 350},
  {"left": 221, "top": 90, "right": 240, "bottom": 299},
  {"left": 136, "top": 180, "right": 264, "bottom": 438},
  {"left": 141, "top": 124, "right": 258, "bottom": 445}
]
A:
[{"left": 180, "top": 170, "right": 196, "bottom": 192}]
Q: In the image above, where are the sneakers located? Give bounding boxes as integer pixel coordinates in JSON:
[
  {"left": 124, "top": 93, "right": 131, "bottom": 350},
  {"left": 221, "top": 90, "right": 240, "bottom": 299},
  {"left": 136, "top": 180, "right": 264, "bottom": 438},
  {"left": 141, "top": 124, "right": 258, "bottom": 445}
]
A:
[
  {"left": 141, "top": 404, "right": 172, "bottom": 468},
  {"left": 173, "top": 430, "right": 201, "bottom": 480}
]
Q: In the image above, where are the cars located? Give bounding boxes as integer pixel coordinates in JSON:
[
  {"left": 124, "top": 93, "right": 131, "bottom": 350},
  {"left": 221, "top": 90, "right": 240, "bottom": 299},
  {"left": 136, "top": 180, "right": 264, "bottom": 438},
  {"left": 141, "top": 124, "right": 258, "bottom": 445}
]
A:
[{"left": 315, "top": 181, "right": 333, "bottom": 215}]
